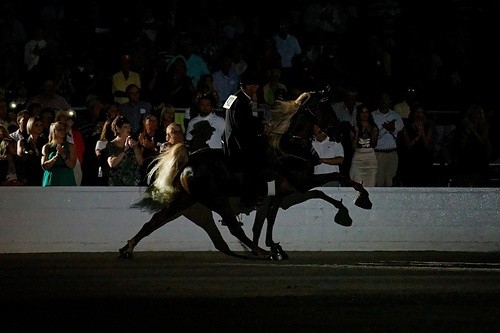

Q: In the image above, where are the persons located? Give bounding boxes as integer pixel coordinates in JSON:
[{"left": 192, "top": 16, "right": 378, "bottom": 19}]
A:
[{"left": 0, "top": 0, "right": 500, "bottom": 200}]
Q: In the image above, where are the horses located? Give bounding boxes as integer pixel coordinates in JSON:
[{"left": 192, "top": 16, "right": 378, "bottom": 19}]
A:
[{"left": 119, "top": 88, "right": 373, "bottom": 261}]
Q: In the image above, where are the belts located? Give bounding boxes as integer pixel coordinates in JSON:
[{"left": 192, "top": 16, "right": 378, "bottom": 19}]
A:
[{"left": 374, "top": 147, "right": 399, "bottom": 153}]
